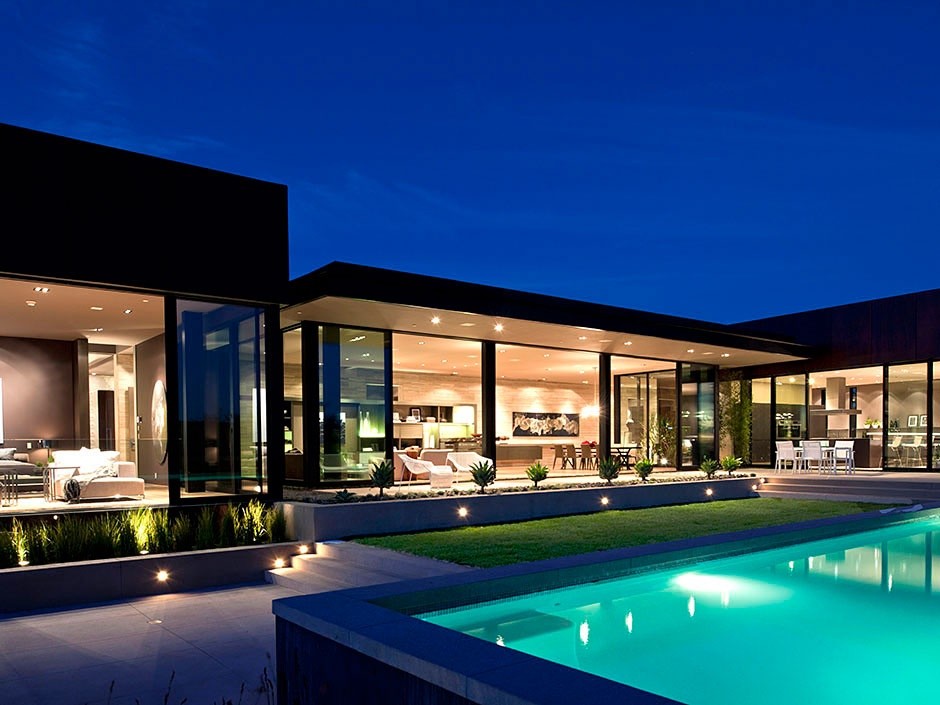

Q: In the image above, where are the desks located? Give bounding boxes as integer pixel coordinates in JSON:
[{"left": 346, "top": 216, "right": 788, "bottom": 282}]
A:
[
  {"left": 550, "top": 445, "right": 644, "bottom": 469},
  {"left": 792, "top": 446, "right": 836, "bottom": 474},
  {"left": 37, "top": 465, "right": 80, "bottom": 502}
]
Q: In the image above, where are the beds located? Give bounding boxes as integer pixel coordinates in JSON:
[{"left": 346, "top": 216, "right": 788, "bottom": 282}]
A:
[{"left": 0, "top": 448, "right": 42, "bottom": 495}]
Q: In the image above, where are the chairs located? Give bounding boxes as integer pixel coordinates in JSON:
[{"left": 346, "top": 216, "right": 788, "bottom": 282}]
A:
[
  {"left": 553, "top": 444, "right": 599, "bottom": 470},
  {"left": 888, "top": 434, "right": 940, "bottom": 467},
  {"left": 392, "top": 446, "right": 497, "bottom": 488},
  {"left": 774, "top": 440, "right": 856, "bottom": 476}
]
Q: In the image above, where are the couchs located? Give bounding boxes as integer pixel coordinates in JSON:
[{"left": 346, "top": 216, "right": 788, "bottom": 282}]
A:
[{"left": 50, "top": 450, "right": 145, "bottom": 502}]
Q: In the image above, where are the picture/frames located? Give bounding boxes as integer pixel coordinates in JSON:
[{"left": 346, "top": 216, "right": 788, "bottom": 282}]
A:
[
  {"left": 907, "top": 415, "right": 919, "bottom": 428},
  {"left": 920, "top": 414, "right": 928, "bottom": 428}
]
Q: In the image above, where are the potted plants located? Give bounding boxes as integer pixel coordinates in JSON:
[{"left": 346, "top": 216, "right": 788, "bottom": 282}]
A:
[
  {"left": 872, "top": 418, "right": 880, "bottom": 429},
  {"left": 863, "top": 417, "right": 872, "bottom": 429}
]
